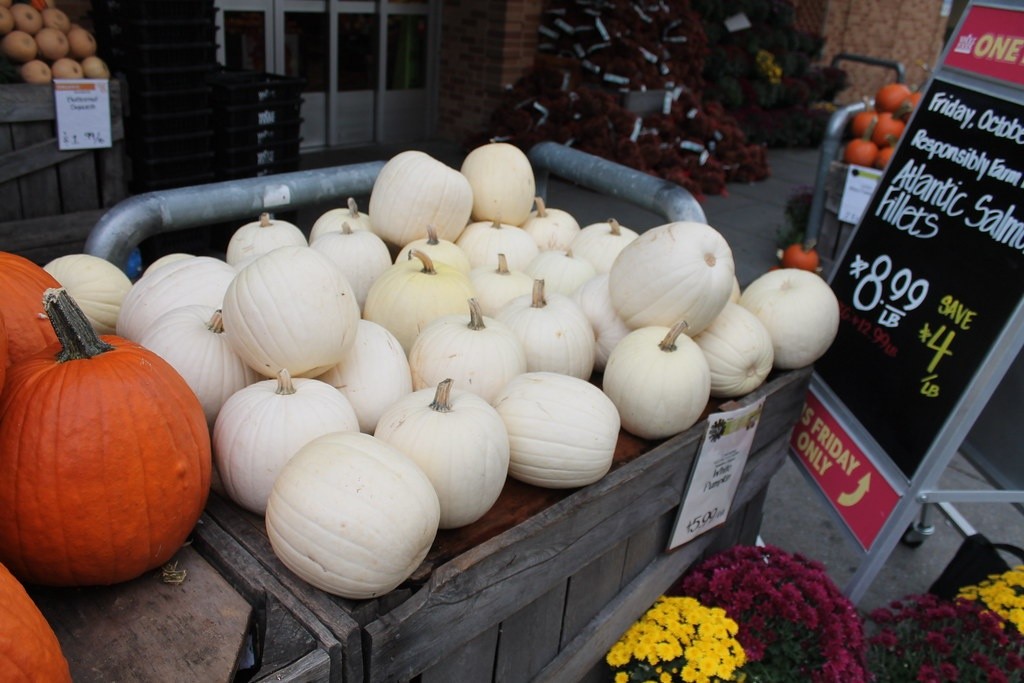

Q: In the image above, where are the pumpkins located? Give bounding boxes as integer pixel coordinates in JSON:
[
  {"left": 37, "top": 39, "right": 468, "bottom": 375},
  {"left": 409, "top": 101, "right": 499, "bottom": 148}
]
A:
[{"left": 0, "top": 0, "right": 932, "bottom": 683}]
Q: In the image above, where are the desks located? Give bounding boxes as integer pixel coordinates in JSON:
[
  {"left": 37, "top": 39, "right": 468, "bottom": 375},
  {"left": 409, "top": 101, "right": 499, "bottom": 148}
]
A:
[{"left": 1, "top": 71, "right": 892, "bottom": 683}]
